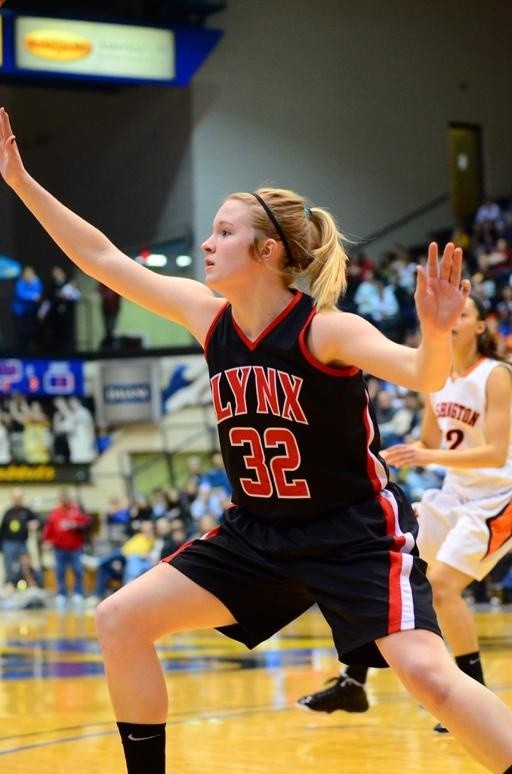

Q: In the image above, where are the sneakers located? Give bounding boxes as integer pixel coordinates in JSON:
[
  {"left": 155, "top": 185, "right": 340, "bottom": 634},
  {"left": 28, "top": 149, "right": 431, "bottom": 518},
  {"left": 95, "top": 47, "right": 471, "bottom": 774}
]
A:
[{"left": 296, "top": 676, "right": 369, "bottom": 712}]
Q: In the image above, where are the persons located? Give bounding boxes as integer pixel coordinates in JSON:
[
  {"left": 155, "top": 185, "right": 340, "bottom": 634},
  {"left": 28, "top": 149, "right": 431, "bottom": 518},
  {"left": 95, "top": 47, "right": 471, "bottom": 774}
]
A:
[
  {"left": 10, "top": 265, "right": 44, "bottom": 359},
  {"left": 9, "top": 393, "right": 56, "bottom": 465},
  {"left": 51, "top": 395, "right": 100, "bottom": 465},
  {"left": 293, "top": 293, "right": 512, "bottom": 734},
  {"left": 37, "top": 266, "right": 76, "bottom": 361},
  {"left": 0, "top": 108, "right": 512, "bottom": 774}
]
